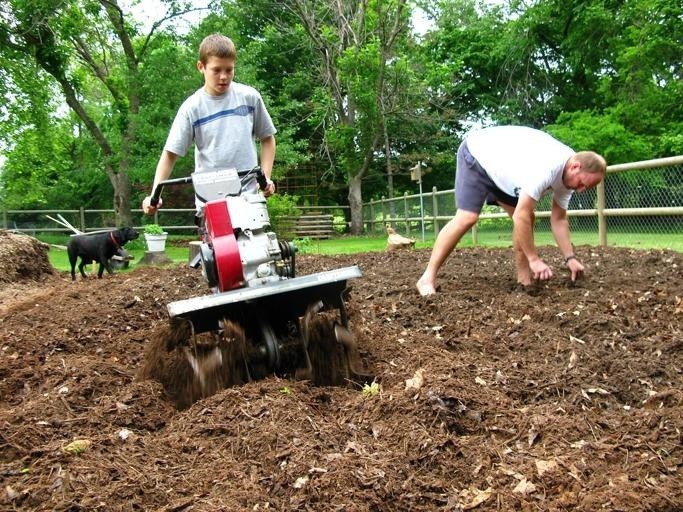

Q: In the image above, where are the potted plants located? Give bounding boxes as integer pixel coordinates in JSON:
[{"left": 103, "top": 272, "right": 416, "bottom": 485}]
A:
[{"left": 143, "top": 223, "right": 168, "bottom": 252}]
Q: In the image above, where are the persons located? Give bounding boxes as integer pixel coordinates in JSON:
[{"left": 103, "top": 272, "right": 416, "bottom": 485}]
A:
[
  {"left": 139, "top": 31, "right": 276, "bottom": 234},
  {"left": 413, "top": 125, "right": 607, "bottom": 300}
]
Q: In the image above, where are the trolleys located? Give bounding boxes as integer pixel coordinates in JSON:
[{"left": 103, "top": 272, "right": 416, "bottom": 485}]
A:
[{"left": 136, "top": 170, "right": 372, "bottom": 390}]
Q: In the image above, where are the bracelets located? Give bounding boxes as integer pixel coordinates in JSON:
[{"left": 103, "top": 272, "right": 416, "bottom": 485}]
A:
[{"left": 562, "top": 256, "right": 577, "bottom": 262}]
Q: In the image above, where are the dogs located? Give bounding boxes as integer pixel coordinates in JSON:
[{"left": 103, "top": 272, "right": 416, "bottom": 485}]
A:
[{"left": 67, "top": 226, "right": 140, "bottom": 281}]
